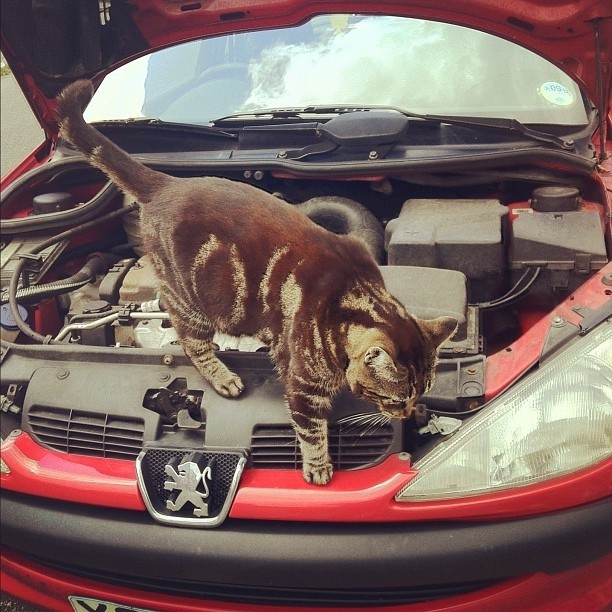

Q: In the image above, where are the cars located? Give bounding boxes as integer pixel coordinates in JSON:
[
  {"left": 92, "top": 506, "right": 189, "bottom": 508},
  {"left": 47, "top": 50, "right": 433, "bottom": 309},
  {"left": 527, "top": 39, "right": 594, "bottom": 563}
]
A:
[{"left": 1, "top": 0, "right": 612, "bottom": 611}]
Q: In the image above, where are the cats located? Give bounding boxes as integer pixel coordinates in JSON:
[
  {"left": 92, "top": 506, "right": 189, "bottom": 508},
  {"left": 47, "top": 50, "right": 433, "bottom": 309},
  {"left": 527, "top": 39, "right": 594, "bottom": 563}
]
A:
[{"left": 52, "top": 77, "right": 459, "bottom": 486}]
